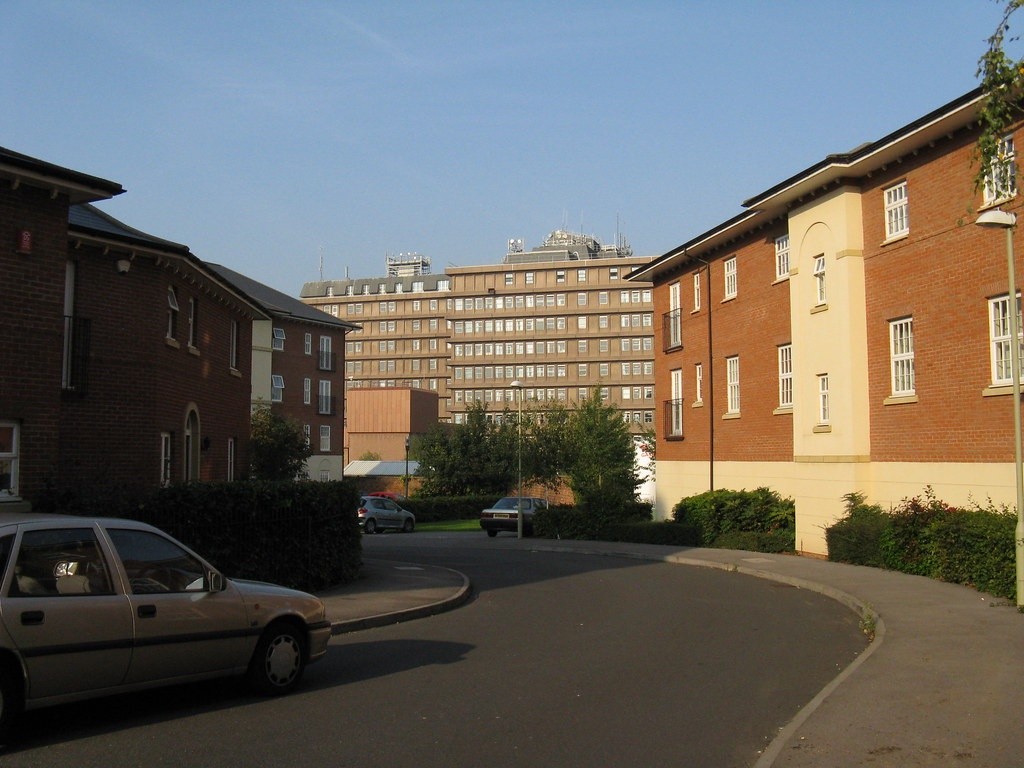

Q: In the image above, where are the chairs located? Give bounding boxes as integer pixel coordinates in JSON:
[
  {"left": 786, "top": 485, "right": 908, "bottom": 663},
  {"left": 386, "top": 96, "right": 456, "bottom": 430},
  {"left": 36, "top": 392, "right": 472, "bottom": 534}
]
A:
[
  {"left": 85, "top": 563, "right": 108, "bottom": 593},
  {"left": 127, "top": 569, "right": 145, "bottom": 595},
  {"left": 14, "top": 565, "right": 47, "bottom": 595},
  {"left": 56, "top": 575, "right": 91, "bottom": 595}
]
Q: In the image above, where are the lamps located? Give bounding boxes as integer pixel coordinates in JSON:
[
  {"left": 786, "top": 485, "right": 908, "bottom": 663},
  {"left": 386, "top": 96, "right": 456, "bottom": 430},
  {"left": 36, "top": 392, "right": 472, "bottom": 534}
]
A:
[{"left": 117, "top": 255, "right": 132, "bottom": 275}]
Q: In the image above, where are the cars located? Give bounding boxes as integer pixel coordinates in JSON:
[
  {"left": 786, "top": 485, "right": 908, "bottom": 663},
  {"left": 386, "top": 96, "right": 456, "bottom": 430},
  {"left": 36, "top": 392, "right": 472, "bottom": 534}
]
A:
[
  {"left": 479, "top": 495, "right": 554, "bottom": 537},
  {"left": 355, "top": 492, "right": 415, "bottom": 519},
  {"left": 0, "top": 512, "right": 334, "bottom": 740},
  {"left": 357, "top": 495, "right": 416, "bottom": 535}
]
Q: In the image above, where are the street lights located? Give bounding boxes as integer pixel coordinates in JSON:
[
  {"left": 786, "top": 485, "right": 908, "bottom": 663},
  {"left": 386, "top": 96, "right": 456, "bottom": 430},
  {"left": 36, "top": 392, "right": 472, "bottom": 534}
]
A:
[
  {"left": 510, "top": 380, "right": 523, "bottom": 540},
  {"left": 404, "top": 436, "right": 410, "bottom": 497}
]
